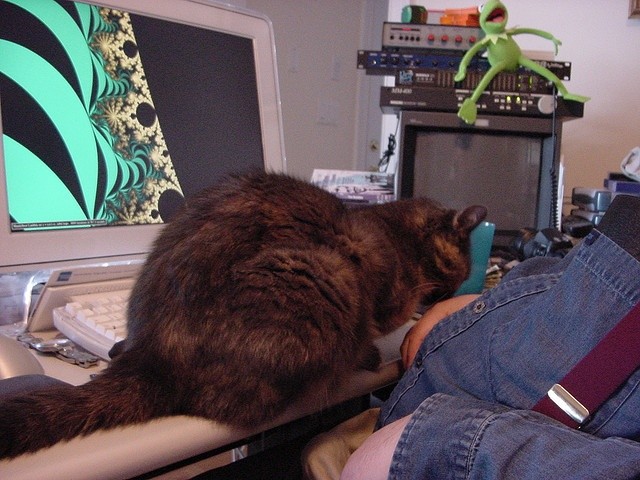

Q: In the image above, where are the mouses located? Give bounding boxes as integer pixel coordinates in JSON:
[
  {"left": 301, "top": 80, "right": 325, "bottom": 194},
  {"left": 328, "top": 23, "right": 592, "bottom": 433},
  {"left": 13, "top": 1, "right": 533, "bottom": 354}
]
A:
[{"left": 0, "top": 334, "right": 45, "bottom": 381}]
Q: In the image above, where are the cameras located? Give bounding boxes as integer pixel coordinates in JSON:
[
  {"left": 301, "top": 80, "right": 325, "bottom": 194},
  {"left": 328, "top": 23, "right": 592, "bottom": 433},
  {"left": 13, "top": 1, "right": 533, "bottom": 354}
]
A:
[{"left": 512, "top": 225, "right": 572, "bottom": 260}]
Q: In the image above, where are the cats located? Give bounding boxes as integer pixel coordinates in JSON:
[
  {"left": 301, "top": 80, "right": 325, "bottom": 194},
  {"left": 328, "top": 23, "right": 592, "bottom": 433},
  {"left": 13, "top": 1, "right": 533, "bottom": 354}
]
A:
[{"left": 1, "top": 168, "right": 488, "bottom": 461}]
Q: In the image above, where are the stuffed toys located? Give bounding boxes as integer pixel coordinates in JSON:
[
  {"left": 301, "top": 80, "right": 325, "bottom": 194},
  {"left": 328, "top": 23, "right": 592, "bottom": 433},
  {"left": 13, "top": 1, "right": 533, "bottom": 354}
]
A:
[{"left": 453, "top": 2, "right": 591, "bottom": 124}]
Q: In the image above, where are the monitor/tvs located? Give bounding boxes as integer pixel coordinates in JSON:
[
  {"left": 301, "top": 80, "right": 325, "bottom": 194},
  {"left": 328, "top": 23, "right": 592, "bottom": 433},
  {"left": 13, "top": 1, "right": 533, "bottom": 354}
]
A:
[
  {"left": 0, "top": 2, "right": 287, "bottom": 274},
  {"left": 396, "top": 111, "right": 564, "bottom": 243}
]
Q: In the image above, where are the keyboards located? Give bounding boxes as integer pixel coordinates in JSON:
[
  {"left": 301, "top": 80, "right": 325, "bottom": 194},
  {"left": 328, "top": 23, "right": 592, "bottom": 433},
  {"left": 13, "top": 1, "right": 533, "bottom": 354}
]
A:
[{"left": 53, "top": 290, "right": 132, "bottom": 363}]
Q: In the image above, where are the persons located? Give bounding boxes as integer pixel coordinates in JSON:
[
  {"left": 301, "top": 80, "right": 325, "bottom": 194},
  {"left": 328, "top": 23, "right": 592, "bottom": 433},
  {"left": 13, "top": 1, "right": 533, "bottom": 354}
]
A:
[{"left": 303, "top": 195, "right": 640, "bottom": 479}]
